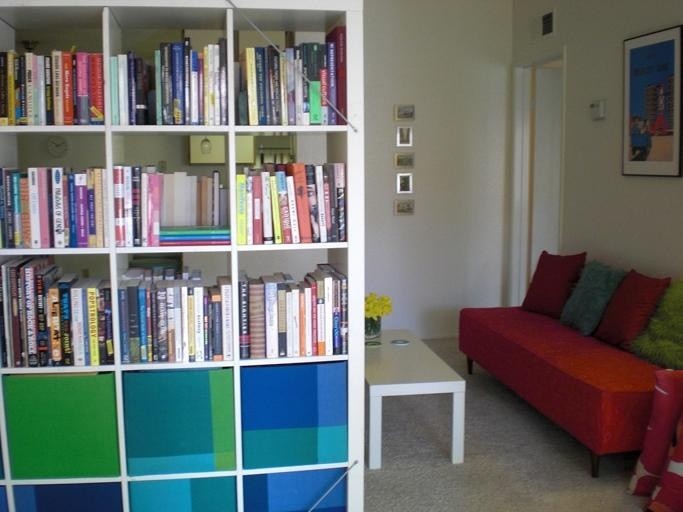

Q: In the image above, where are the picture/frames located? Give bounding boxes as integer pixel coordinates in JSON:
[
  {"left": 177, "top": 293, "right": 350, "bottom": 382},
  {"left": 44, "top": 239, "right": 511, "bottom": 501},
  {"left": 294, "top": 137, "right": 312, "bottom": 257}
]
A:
[
  {"left": 396, "top": 172, "right": 414, "bottom": 194},
  {"left": 394, "top": 103, "right": 415, "bottom": 121},
  {"left": 394, "top": 198, "right": 416, "bottom": 215},
  {"left": 396, "top": 126, "right": 413, "bottom": 147},
  {"left": 394, "top": 152, "right": 415, "bottom": 169},
  {"left": 622, "top": 24, "right": 683, "bottom": 177}
]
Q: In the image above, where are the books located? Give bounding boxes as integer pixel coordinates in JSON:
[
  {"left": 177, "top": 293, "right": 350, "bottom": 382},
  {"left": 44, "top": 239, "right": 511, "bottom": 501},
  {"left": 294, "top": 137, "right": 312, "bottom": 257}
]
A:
[
  {"left": 108, "top": 36, "right": 229, "bottom": 126},
  {"left": 0, "top": 49, "right": 105, "bottom": 126},
  {"left": 235, "top": 161, "right": 347, "bottom": 246},
  {"left": 118, "top": 253, "right": 234, "bottom": 366},
  {"left": 0, "top": 165, "right": 110, "bottom": 249},
  {"left": 238, "top": 263, "right": 349, "bottom": 361},
  {"left": 113, "top": 162, "right": 231, "bottom": 248},
  {"left": 233, "top": 26, "right": 348, "bottom": 127},
  {"left": 0, "top": 255, "right": 115, "bottom": 368}
]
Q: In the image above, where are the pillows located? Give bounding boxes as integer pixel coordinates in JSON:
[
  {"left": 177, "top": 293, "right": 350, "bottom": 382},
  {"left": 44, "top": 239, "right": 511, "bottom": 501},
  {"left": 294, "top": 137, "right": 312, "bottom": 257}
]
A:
[
  {"left": 630, "top": 279, "right": 683, "bottom": 369},
  {"left": 560, "top": 260, "right": 626, "bottom": 336},
  {"left": 597, "top": 269, "right": 671, "bottom": 347},
  {"left": 521, "top": 250, "right": 586, "bottom": 318},
  {"left": 624, "top": 370, "right": 683, "bottom": 496},
  {"left": 644, "top": 416, "right": 683, "bottom": 512}
]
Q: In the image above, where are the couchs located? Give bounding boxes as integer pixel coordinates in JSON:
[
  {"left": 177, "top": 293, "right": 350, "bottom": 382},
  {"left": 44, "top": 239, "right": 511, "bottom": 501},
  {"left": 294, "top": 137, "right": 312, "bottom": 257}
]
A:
[{"left": 459, "top": 251, "right": 682, "bottom": 478}]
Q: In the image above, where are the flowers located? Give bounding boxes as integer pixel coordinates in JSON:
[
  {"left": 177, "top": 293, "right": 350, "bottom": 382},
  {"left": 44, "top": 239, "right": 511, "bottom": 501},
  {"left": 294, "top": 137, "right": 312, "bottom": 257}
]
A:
[{"left": 365, "top": 292, "right": 393, "bottom": 336}]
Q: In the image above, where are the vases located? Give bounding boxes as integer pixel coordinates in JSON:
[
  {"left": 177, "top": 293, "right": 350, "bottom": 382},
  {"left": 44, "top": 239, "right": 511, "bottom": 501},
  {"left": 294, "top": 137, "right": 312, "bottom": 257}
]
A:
[{"left": 365, "top": 316, "right": 382, "bottom": 339}]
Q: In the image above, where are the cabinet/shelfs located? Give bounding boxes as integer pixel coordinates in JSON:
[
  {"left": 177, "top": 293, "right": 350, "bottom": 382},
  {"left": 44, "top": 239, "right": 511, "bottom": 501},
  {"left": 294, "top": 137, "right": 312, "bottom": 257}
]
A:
[{"left": 1, "top": 1, "right": 366, "bottom": 512}]
